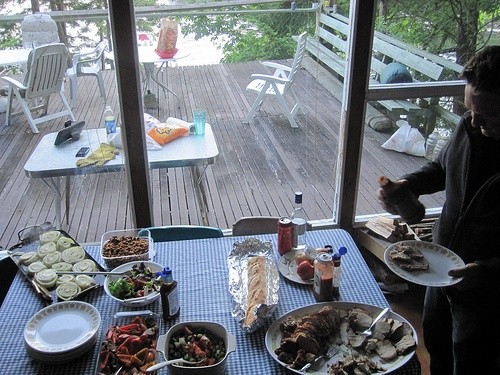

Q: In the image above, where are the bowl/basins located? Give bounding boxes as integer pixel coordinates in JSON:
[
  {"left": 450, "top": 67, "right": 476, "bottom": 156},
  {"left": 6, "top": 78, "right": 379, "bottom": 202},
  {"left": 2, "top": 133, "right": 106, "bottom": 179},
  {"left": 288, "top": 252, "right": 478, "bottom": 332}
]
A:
[
  {"left": 104, "top": 261, "right": 165, "bottom": 307},
  {"left": 154, "top": 47, "right": 178, "bottom": 58},
  {"left": 155, "top": 322, "right": 237, "bottom": 375},
  {"left": 94, "top": 310, "right": 162, "bottom": 375},
  {"left": 100, "top": 229, "right": 152, "bottom": 269}
]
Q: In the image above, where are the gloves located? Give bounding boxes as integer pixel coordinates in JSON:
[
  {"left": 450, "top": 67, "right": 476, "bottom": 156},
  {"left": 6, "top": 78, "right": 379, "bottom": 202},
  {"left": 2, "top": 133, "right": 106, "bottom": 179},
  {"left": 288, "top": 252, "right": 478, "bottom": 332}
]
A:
[{"left": 76, "top": 143, "right": 120, "bottom": 168}]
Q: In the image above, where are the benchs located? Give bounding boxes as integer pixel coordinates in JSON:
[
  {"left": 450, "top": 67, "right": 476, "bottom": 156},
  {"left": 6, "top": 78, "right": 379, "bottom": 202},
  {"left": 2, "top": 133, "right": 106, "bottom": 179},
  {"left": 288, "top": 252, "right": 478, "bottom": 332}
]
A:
[{"left": 292, "top": 13, "right": 444, "bottom": 128}]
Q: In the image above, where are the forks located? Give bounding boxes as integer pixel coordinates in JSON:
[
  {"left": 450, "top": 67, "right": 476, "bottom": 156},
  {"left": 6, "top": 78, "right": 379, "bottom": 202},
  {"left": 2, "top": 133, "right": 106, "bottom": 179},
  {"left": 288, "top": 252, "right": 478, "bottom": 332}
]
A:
[
  {"left": 362, "top": 306, "right": 392, "bottom": 337},
  {"left": 299, "top": 347, "right": 339, "bottom": 372}
]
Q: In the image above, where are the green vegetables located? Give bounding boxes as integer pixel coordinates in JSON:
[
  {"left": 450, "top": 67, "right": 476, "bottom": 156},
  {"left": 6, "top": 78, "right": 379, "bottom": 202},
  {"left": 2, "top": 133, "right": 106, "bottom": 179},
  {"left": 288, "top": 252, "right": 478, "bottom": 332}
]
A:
[{"left": 168, "top": 326, "right": 226, "bottom": 366}]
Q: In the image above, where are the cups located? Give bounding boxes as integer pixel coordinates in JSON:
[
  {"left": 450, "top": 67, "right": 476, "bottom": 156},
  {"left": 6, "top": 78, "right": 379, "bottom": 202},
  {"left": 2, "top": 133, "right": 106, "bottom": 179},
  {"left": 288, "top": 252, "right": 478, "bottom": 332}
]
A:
[{"left": 192, "top": 109, "right": 206, "bottom": 136}]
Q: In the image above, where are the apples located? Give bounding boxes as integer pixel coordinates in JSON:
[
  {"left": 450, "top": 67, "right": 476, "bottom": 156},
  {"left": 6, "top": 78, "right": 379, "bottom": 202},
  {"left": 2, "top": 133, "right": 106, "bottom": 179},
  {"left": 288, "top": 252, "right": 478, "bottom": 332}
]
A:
[{"left": 297, "top": 261, "right": 315, "bottom": 280}]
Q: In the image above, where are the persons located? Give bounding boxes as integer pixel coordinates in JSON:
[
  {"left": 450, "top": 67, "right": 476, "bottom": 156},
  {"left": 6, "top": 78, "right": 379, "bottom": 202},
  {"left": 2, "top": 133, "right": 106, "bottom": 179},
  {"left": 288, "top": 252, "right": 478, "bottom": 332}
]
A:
[{"left": 377, "top": 45, "right": 500, "bottom": 375}]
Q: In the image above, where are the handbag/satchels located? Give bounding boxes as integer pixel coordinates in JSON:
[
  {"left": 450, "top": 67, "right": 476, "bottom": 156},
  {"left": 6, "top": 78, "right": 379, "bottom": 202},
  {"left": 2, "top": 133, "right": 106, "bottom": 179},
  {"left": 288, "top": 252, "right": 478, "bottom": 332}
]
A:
[
  {"left": 157, "top": 18, "right": 178, "bottom": 52},
  {"left": 381, "top": 120, "right": 426, "bottom": 157}
]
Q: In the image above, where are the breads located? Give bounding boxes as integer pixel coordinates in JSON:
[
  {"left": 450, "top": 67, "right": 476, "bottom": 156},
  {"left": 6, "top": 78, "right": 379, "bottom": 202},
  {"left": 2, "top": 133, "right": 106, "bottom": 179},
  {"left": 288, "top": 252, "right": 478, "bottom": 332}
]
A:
[
  {"left": 396, "top": 248, "right": 428, "bottom": 270},
  {"left": 275, "top": 306, "right": 348, "bottom": 356},
  {"left": 346, "top": 308, "right": 416, "bottom": 359}
]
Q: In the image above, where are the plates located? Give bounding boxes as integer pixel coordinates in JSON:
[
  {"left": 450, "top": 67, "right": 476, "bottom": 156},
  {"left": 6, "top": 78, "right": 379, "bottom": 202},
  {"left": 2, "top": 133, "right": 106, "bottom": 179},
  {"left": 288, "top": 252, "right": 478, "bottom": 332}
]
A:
[
  {"left": 278, "top": 250, "right": 314, "bottom": 285},
  {"left": 23, "top": 300, "right": 101, "bottom": 365},
  {"left": 384, "top": 240, "right": 466, "bottom": 287},
  {"left": 264, "top": 301, "right": 418, "bottom": 375},
  {"left": 7, "top": 224, "right": 107, "bottom": 306}
]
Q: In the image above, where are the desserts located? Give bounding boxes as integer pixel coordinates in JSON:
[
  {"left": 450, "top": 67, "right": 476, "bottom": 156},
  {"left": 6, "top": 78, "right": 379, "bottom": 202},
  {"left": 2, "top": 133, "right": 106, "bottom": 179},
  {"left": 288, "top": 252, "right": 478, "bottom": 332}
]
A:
[{"left": 18, "top": 231, "right": 96, "bottom": 301}]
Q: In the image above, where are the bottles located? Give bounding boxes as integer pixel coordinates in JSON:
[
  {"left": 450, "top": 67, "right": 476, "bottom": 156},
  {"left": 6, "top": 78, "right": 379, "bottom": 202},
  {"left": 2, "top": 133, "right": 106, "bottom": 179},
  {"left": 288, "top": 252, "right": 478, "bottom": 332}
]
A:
[
  {"left": 290, "top": 192, "right": 307, "bottom": 250},
  {"left": 332, "top": 247, "right": 347, "bottom": 297},
  {"left": 144, "top": 89, "right": 157, "bottom": 108},
  {"left": 304, "top": 246, "right": 334, "bottom": 302},
  {"left": 424, "top": 130, "right": 450, "bottom": 161},
  {"left": 103, "top": 106, "right": 116, "bottom": 137},
  {"left": 155, "top": 266, "right": 181, "bottom": 321},
  {"left": 378, "top": 175, "right": 426, "bottom": 224}
]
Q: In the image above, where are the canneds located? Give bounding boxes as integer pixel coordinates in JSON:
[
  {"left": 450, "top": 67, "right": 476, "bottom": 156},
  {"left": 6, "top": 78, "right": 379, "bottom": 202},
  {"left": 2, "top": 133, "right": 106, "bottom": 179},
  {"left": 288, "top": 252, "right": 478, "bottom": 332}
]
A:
[{"left": 277, "top": 216, "right": 293, "bottom": 254}]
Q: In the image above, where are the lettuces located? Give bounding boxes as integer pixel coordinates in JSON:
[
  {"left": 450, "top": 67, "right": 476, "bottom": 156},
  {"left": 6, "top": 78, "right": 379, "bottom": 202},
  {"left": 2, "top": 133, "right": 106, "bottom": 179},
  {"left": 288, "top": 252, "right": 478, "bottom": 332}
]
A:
[{"left": 108, "top": 269, "right": 160, "bottom": 299}]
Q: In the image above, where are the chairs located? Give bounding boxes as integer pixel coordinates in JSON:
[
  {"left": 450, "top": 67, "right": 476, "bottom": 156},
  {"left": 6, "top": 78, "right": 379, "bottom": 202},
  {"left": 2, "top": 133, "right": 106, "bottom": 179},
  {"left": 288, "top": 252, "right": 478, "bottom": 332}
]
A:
[
  {"left": 242, "top": 31, "right": 307, "bottom": 128},
  {"left": 66, "top": 40, "right": 108, "bottom": 108},
  {"left": 1, "top": 15, "right": 75, "bottom": 134}
]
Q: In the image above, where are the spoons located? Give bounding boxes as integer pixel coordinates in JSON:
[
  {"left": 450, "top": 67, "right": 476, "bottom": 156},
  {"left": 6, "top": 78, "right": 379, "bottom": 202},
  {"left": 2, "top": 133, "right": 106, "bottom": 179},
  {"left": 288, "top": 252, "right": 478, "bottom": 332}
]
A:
[{"left": 145, "top": 357, "right": 207, "bottom": 372}]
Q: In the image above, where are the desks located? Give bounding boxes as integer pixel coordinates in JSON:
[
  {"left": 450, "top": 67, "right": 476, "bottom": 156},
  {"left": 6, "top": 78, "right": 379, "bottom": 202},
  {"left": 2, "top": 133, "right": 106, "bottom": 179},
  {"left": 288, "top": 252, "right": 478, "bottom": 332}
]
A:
[
  {"left": 0, "top": 229, "right": 421, "bottom": 375},
  {"left": 107, "top": 46, "right": 192, "bottom": 109},
  {"left": 0, "top": 50, "right": 31, "bottom": 65},
  {"left": 24, "top": 122, "right": 219, "bottom": 230}
]
